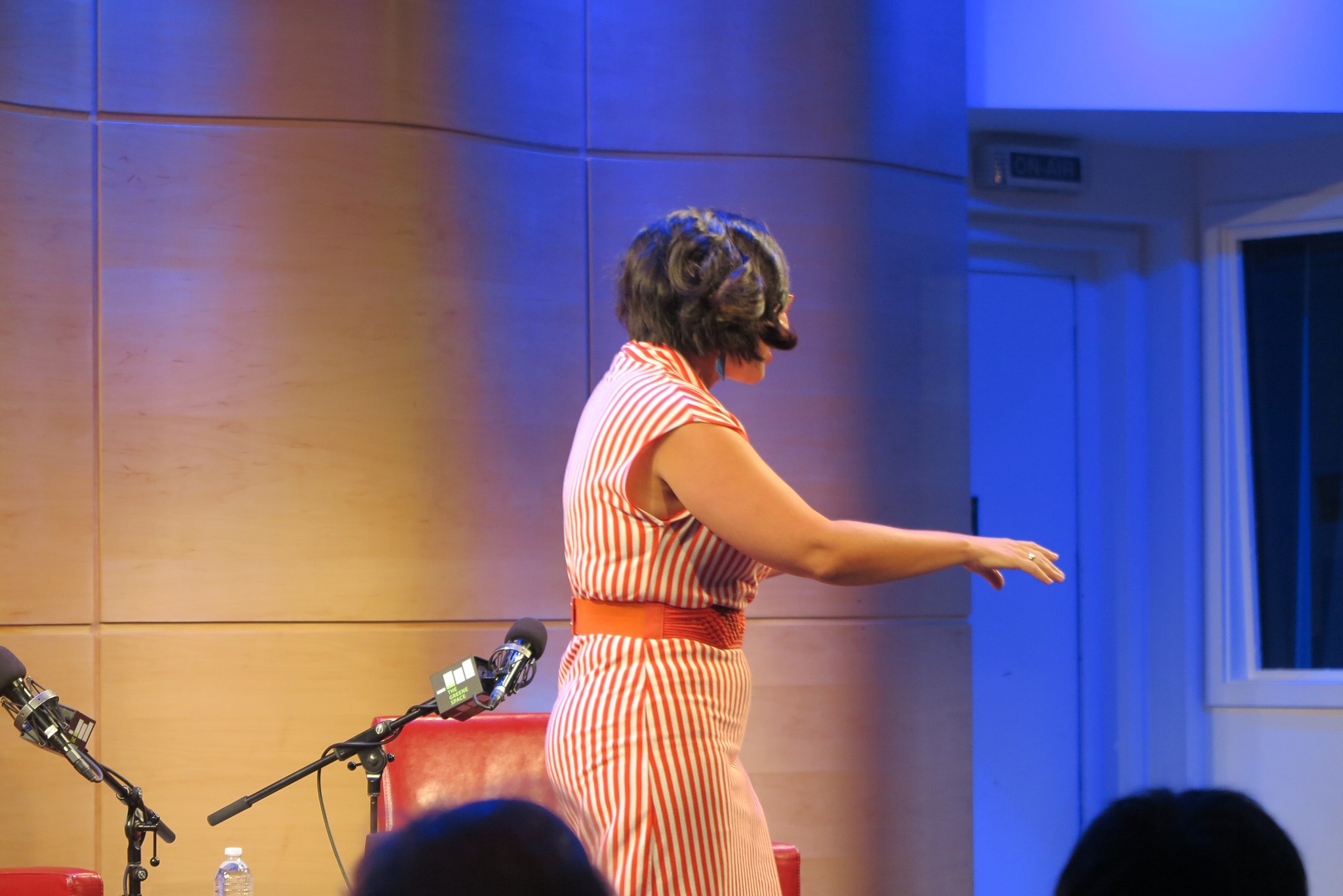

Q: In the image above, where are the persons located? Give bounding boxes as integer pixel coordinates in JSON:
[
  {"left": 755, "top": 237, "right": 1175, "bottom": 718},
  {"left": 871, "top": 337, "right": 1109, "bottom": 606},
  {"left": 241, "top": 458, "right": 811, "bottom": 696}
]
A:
[
  {"left": 352, "top": 799, "right": 614, "bottom": 896},
  {"left": 1054, "top": 788, "right": 1308, "bottom": 896},
  {"left": 544, "top": 207, "right": 1065, "bottom": 896}
]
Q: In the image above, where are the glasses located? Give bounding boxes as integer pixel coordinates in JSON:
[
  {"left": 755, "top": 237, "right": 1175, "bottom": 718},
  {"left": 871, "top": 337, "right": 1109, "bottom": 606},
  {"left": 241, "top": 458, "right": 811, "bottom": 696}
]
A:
[{"left": 775, "top": 293, "right": 793, "bottom": 314}]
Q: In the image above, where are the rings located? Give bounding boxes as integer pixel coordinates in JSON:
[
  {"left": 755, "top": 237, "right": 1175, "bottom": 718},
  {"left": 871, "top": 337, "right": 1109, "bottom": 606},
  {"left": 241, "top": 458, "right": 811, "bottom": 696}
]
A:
[{"left": 1028, "top": 553, "right": 1036, "bottom": 560}]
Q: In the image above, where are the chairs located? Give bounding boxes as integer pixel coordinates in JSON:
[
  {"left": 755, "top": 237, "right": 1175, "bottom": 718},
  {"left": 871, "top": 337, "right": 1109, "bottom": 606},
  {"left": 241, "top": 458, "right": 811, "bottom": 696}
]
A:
[{"left": 373, "top": 715, "right": 801, "bottom": 896}]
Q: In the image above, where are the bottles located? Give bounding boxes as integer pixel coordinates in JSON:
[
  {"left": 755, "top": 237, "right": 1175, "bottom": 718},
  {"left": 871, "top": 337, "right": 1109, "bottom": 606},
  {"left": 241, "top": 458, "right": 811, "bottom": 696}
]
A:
[{"left": 213, "top": 847, "right": 254, "bottom": 896}]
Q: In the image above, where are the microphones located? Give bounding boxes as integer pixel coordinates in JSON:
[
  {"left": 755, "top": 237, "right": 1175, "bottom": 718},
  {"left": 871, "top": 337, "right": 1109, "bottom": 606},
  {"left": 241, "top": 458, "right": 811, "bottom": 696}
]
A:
[
  {"left": 488, "top": 617, "right": 548, "bottom": 710},
  {"left": 0, "top": 645, "right": 98, "bottom": 783}
]
